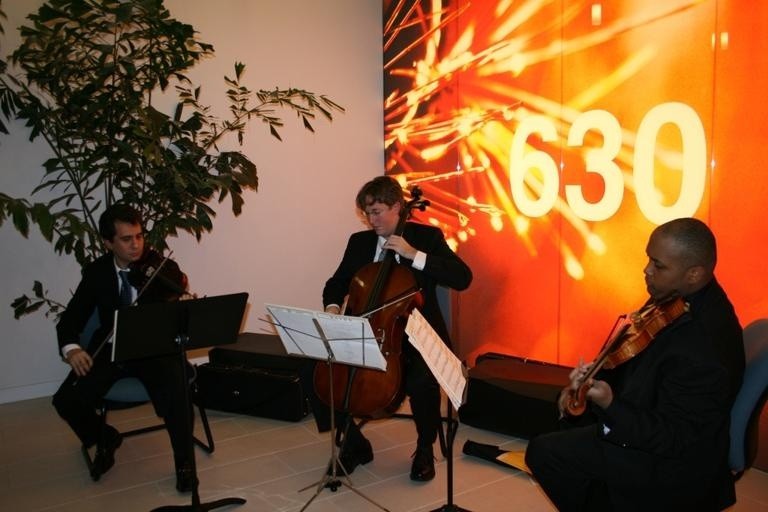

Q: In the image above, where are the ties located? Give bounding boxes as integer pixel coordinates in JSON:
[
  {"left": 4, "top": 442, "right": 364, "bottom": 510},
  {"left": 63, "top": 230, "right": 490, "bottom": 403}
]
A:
[
  {"left": 119, "top": 270, "right": 132, "bottom": 306},
  {"left": 378, "top": 250, "right": 387, "bottom": 262}
]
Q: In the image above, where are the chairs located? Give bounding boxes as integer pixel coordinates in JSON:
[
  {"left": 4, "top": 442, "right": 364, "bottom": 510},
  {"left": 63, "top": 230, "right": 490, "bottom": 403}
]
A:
[
  {"left": 727, "top": 318, "right": 768, "bottom": 480},
  {"left": 82, "top": 351, "right": 214, "bottom": 479},
  {"left": 335, "top": 284, "right": 459, "bottom": 456}
]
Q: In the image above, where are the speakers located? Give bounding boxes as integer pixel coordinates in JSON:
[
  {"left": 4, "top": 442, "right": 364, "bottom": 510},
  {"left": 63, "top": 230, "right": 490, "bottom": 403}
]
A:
[
  {"left": 467, "top": 357, "right": 576, "bottom": 438},
  {"left": 205, "top": 333, "right": 318, "bottom": 423}
]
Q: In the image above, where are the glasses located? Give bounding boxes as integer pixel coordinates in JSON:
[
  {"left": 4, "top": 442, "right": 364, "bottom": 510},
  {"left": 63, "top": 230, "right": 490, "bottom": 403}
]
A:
[{"left": 362, "top": 209, "right": 387, "bottom": 217}]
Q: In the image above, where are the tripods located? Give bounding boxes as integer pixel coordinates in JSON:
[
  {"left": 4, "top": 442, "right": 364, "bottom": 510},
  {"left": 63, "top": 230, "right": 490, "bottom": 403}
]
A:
[
  {"left": 148, "top": 342, "right": 247, "bottom": 512},
  {"left": 298, "top": 354, "right": 391, "bottom": 512}
]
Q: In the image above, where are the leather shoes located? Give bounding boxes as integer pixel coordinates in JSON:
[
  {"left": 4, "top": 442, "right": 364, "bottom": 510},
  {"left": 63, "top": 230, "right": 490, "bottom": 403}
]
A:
[
  {"left": 410, "top": 445, "right": 435, "bottom": 482},
  {"left": 327, "top": 438, "right": 374, "bottom": 477},
  {"left": 93, "top": 424, "right": 123, "bottom": 475},
  {"left": 176, "top": 476, "right": 193, "bottom": 492}
]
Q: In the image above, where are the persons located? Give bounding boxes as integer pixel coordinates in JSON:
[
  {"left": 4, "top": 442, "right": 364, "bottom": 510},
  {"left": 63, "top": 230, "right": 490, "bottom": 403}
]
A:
[
  {"left": 51, "top": 202, "right": 203, "bottom": 494},
  {"left": 298, "top": 175, "right": 474, "bottom": 481},
  {"left": 524, "top": 217, "right": 747, "bottom": 510}
]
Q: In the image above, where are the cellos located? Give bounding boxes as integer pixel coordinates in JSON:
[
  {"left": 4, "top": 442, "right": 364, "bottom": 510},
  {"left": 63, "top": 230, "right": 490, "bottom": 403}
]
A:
[{"left": 313, "top": 186, "right": 429, "bottom": 418}]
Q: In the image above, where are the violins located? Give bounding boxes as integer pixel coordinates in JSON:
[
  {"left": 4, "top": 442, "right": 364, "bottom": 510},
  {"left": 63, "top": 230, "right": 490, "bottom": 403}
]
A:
[
  {"left": 567, "top": 289, "right": 689, "bottom": 416},
  {"left": 126, "top": 251, "right": 198, "bottom": 301}
]
